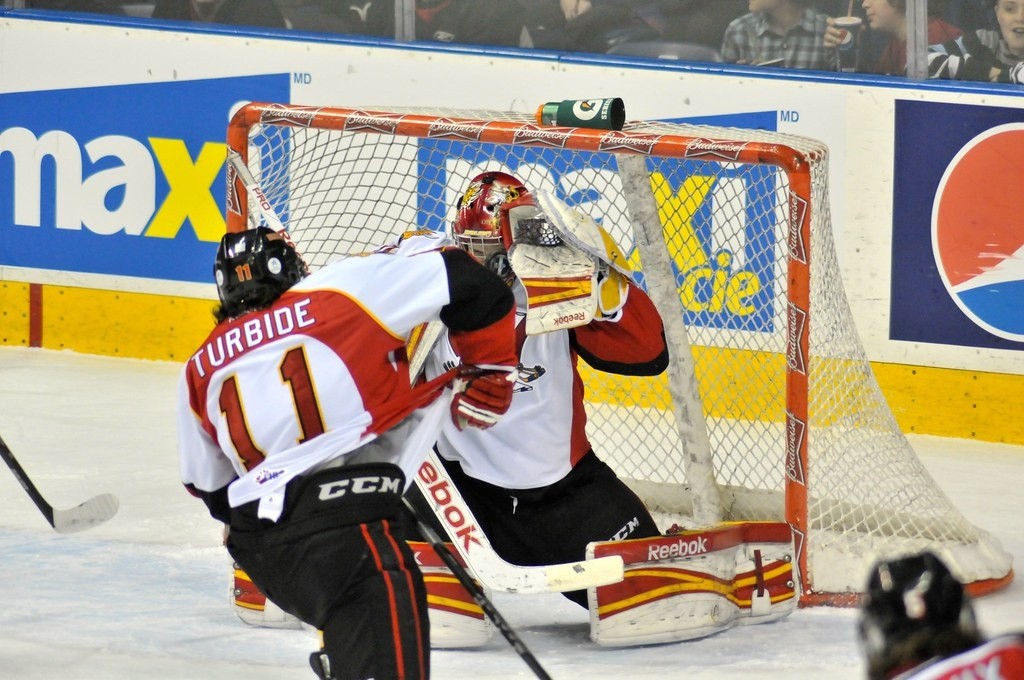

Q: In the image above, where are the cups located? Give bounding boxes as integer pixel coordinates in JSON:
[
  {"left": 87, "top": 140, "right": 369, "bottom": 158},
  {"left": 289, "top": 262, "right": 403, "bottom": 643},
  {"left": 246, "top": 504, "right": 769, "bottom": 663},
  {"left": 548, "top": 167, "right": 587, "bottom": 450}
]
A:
[{"left": 834, "top": 16, "right": 862, "bottom": 72}]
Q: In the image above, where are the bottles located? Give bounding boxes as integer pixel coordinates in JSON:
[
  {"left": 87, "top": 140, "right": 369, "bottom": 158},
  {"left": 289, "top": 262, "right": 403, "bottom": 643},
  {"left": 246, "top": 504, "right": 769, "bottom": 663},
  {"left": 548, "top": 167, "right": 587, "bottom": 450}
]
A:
[{"left": 533, "top": 97, "right": 626, "bottom": 131}]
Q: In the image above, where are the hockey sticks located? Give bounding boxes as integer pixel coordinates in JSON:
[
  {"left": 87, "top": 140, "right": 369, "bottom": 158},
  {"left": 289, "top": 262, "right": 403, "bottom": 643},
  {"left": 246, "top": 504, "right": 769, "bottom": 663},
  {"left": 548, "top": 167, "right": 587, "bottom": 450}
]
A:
[
  {"left": 397, "top": 496, "right": 552, "bottom": 680},
  {"left": 226, "top": 147, "right": 625, "bottom": 593},
  {"left": 0, "top": 437, "right": 119, "bottom": 534}
]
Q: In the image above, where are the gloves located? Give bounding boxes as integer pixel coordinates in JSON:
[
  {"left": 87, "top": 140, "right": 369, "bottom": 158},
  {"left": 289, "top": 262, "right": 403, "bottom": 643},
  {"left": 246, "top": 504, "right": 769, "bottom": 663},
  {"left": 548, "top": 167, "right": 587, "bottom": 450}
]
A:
[{"left": 448, "top": 296, "right": 519, "bottom": 431}]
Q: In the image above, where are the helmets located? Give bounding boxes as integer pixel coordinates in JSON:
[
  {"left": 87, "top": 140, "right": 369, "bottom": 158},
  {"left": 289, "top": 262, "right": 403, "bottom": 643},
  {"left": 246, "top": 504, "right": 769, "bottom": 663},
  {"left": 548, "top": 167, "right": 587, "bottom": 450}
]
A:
[
  {"left": 454, "top": 172, "right": 529, "bottom": 242},
  {"left": 213, "top": 227, "right": 305, "bottom": 315},
  {"left": 860, "top": 552, "right": 983, "bottom": 679}
]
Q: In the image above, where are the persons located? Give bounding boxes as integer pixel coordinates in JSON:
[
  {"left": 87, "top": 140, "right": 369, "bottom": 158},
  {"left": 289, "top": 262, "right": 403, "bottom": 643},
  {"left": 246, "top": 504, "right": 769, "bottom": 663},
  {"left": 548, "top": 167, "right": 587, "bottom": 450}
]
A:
[
  {"left": 0, "top": 1, "right": 1022, "bottom": 94},
  {"left": 179, "top": 224, "right": 520, "bottom": 679},
  {"left": 228, "top": 166, "right": 797, "bottom": 649},
  {"left": 855, "top": 546, "right": 1023, "bottom": 679}
]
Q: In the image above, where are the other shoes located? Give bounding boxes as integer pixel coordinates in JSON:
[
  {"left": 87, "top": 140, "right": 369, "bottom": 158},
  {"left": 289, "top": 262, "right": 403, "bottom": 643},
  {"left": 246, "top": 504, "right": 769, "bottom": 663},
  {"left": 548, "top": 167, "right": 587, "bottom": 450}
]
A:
[{"left": 310, "top": 650, "right": 332, "bottom": 680}]
